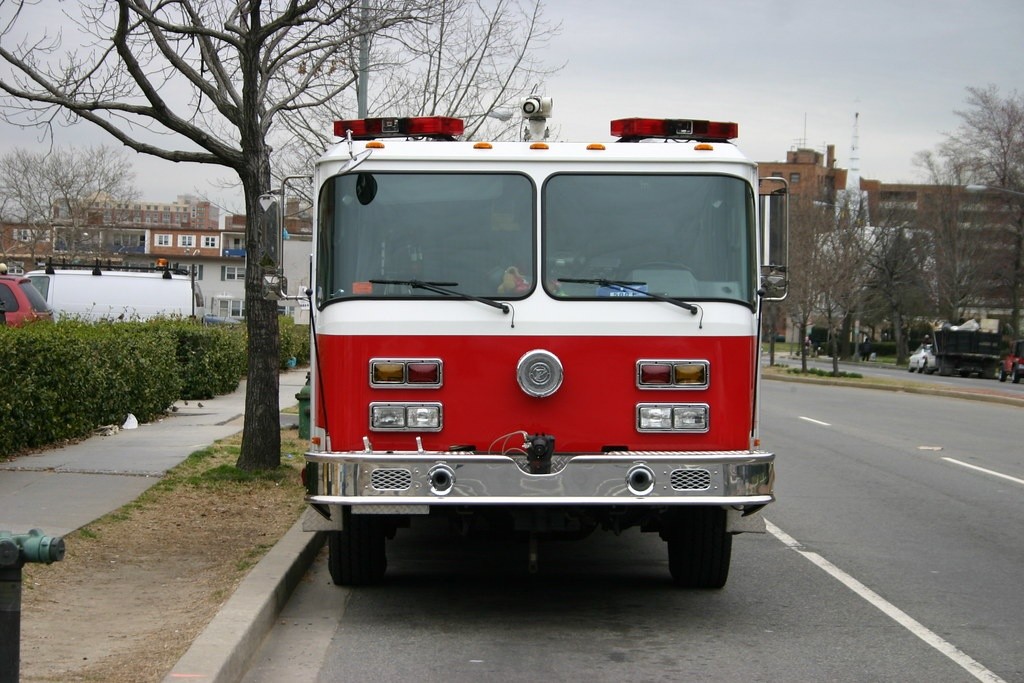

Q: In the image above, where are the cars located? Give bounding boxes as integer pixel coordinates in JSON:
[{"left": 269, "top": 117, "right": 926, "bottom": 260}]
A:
[
  {"left": 907, "top": 343, "right": 937, "bottom": 374},
  {"left": 0, "top": 274, "right": 56, "bottom": 330}
]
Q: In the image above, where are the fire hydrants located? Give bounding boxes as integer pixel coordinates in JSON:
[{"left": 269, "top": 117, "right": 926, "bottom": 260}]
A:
[{"left": 295, "top": 372, "right": 311, "bottom": 440}]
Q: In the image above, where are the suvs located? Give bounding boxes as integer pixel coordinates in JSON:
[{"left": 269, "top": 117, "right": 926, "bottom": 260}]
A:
[{"left": 997, "top": 339, "right": 1024, "bottom": 383}]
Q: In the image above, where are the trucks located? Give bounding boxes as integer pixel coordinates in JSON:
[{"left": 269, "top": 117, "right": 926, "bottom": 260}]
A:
[
  {"left": 18, "top": 263, "right": 204, "bottom": 328},
  {"left": 932, "top": 326, "right": 1008, "bottom": 379}
]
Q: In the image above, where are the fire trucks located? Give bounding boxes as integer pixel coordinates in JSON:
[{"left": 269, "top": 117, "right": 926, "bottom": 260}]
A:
[{"left": 253, "top": 87, "right": 790, "bottom": 590}]
